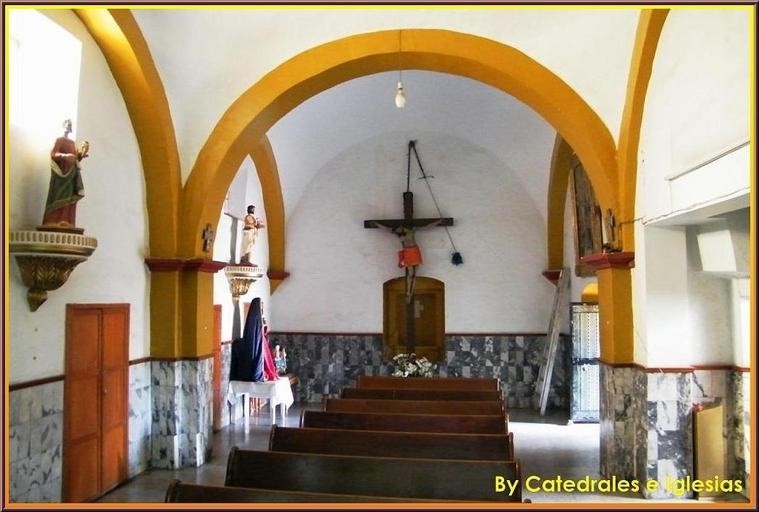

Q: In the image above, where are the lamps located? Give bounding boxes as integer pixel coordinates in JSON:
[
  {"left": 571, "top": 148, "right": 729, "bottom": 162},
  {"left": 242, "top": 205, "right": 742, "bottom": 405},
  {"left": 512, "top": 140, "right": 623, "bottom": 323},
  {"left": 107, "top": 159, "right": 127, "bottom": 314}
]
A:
[{"left": 395, "top": 28, "right": 412, "bottom": 110}]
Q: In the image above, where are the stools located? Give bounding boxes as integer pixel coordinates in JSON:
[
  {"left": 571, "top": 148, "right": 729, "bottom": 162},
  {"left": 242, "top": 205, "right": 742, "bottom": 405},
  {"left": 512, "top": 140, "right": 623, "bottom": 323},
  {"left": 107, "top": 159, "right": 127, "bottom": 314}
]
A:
[{"left": 229, "top": 377, "right": 294, "bottom": 433}]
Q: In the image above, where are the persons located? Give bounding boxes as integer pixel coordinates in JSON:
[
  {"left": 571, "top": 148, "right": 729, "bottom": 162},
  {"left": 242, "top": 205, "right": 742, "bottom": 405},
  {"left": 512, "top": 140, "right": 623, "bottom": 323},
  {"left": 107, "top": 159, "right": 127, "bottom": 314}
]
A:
[
  {"left": 42, "top": 118, "right": 89, "bottom": 229},
  {"left": 372, "top": 219, "right": 449, "bottom": 303},
  {"left": 239, "top": 204, "right": 265, "bottom": 267}
]
